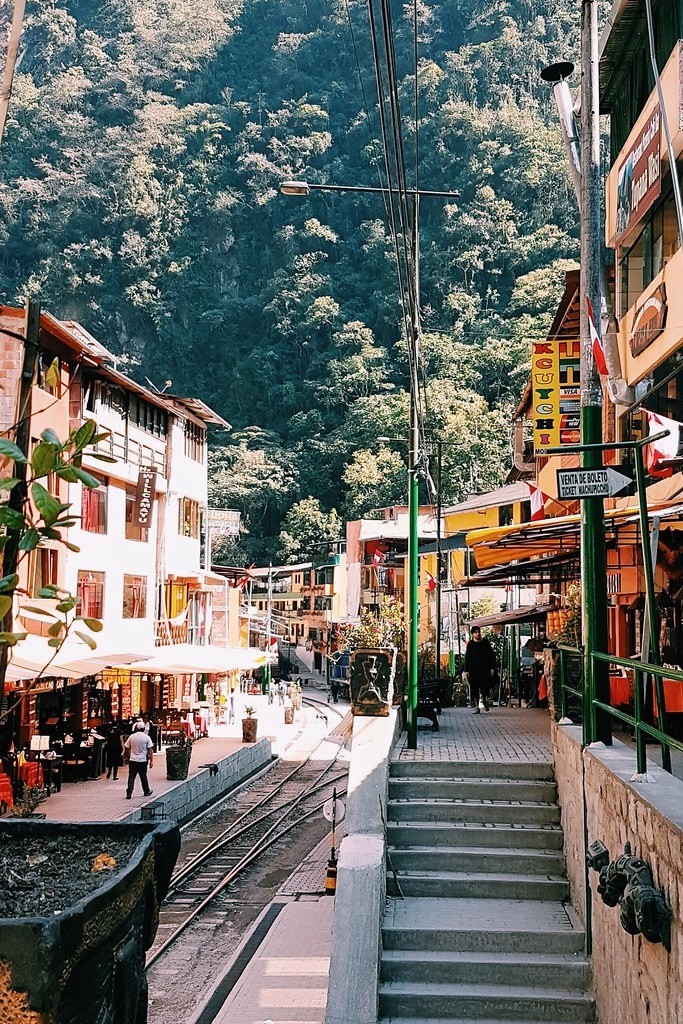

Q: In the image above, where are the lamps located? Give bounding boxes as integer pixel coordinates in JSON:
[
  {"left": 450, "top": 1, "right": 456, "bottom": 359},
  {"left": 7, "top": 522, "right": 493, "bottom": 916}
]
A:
[{"left": 97, "top": 676, "right": 119, "bottom": 691}]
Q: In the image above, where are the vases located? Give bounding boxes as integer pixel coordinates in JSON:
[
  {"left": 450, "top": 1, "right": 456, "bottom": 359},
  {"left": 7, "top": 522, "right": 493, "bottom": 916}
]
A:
[{"left": 349, "top": 646, "right": 407, "bottom": 716}]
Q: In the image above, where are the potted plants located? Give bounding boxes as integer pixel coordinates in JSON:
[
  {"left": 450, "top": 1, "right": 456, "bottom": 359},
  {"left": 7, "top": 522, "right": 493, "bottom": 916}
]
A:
[
  {"left": 242, "top": 704, "right": 257, "bottom": 742},
  {"left": 0, "top": 344, "right": 180, "bottom": 1024},
  {"left": 165, "top": 725, "right": 192, "bottom": 780}
]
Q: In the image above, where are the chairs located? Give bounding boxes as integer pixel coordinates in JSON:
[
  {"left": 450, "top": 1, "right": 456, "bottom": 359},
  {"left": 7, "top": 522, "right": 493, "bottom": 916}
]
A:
[
  {"left": 1, "top": 751, "right": 23, "bottom": 806},
  {"left": 401, "top": 666, "right": 442, "bottom": 731}
]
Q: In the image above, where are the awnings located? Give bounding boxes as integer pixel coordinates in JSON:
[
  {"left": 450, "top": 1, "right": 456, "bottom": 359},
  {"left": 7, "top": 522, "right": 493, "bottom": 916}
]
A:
[
  {"left": 114, "top": 643, "right": 279, "bottom": 674},
  {"left": 464, "top": 604, "right": 558, "bottom": 627},
  {"left": 458, "top": 498, "right": 683, "bottom": 588},
  {"left": 4, "top": 634, "right": 154, "bottom": 683}
]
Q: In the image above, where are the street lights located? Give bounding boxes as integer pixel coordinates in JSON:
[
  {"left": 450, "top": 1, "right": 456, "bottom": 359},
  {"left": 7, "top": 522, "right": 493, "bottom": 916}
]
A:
[
  {"left": 375, "top": 435, "right": 464, "bottom": 679},
  {"left": 279, "top": 180, "right": 460, "bottom": 751},
  {"left": 267, "top": 561, "right": 279, "bottom": 705}
]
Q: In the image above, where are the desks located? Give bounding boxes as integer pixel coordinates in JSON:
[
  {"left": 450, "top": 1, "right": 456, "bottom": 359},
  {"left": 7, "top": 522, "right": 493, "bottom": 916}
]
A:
[{"left": 0, "top": 708, "right": 208, "bottom": 817}]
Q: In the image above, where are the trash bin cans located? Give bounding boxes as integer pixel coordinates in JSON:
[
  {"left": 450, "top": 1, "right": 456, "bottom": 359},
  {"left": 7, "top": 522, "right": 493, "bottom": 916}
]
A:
[
  {"left": 165, "top": 746, "right": 192, "bottom": 781},
  {"left": 334, "top": 649, "right": 350, "bottom": 678},
  {"left": 548, "top": 653, "right": 583, "bottom": 724}
]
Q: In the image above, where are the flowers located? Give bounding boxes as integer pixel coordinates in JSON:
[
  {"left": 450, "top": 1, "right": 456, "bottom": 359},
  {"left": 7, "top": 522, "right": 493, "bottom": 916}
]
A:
[{"left": 325, "top": 595, "right": 411, "bottom": 660}]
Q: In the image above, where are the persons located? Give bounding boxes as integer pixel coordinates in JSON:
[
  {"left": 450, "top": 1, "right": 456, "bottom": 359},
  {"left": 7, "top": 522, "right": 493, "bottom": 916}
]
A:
[
  {"left": 330, "top": 680, "right": 339, "bottom": 704},
  {"left": 35, "top": 678, "right": 288, "bottom": 799},
  {"left": 464, "top": 626, "right": 496, "bottom": 714}
]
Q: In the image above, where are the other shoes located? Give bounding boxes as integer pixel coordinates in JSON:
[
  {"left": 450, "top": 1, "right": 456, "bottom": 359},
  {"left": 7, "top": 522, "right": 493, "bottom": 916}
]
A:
[
  {"left": 125, "top": 793, "right": 131, "bottom": 799},
  {"left": 106, "top": 771, "right": 111, "bottom": 779},
  {"left": 484, "top": 705, "right": 489, "bottom": 711},
  {"left": 144, "top": 790, "right": 153, "bottom": 796},
  {"left": 472, "top": 707, "right": 480, "bottom": 714},
  {"left": 113, "top": 777, "right": 119, "bottom": 780}
]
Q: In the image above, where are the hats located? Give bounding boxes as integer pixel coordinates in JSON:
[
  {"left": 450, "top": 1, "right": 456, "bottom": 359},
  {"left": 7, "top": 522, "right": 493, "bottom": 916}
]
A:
[
  {"left": 136, "top": 718, "right": 144, "bottom": 722},
  {"left": 137, "top": 721, "right": 145, "bottom": 729}
]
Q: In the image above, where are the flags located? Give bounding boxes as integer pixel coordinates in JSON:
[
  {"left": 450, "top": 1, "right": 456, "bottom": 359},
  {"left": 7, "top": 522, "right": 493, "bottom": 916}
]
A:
[
  {"left": 529, "top": 486, "right": 549, "bottom": 521},
  {"left": 265, "top": 638, "right": 278, "bottom": 653},
  {"left": 647, "top": 413, "right": 680, "bottom": 477},
  {"left": 373, "top": 549, "right": 382, "bottom": 565},
  {"left": 427, "top": 573, "right": 437, "bottom": 591}
]
